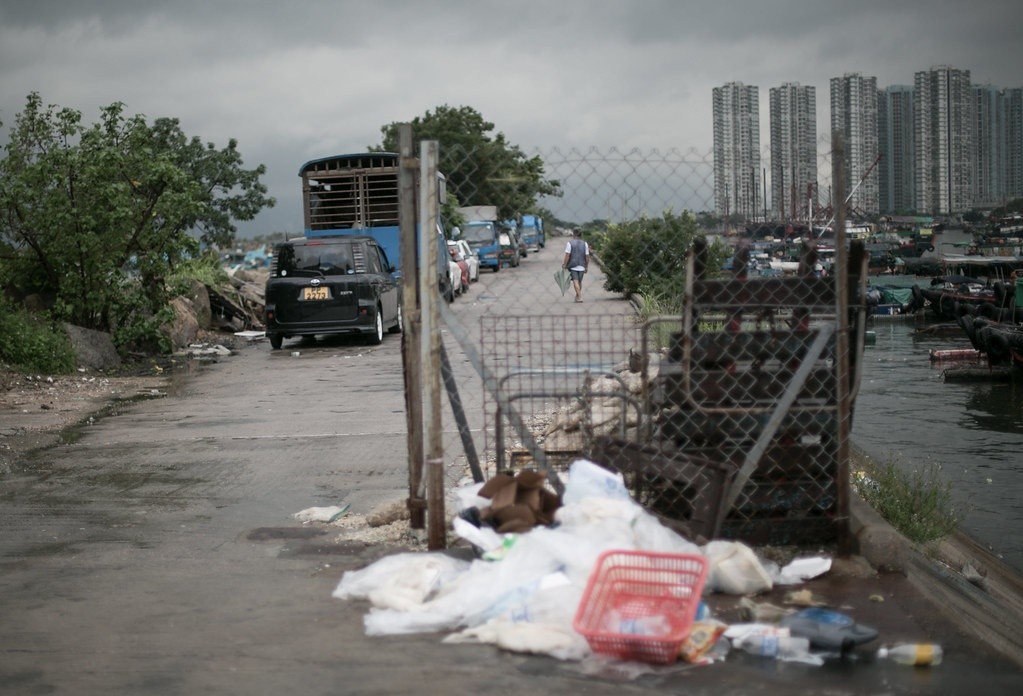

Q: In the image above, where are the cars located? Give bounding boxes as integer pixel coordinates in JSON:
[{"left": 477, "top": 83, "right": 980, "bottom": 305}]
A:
[{"left": 446, "top": 240, "right": 480, "bottom": 298}]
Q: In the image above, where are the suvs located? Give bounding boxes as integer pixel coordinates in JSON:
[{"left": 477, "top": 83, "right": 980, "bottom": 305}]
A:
[{"left": 265, "top": 235, "right": 403, "bottom": 350}]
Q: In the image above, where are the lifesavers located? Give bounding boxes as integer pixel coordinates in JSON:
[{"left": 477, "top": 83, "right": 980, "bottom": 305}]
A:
[
  {"left": 992, "top": 281, "right": 1005, "bottom": 298},
  {"left": 928, "top": 244, "right": 935, "bottom": 253},
  {"left": 962, "top": 315, "right": 987, "bottom": 351},
  {"left": 912, "top": 284, "right": 923, "bottom": 309},
  {"left": 986, "top": 333, "right": 1008, "bottom": 357},
  {"left": 787, "top": 224, "right": 793, "bottom": 234},
  {"left": 979, "top": 303, "right": 996, "bottom": 320}
]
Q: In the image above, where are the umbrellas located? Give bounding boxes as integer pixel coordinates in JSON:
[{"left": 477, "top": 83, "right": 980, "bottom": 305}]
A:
[{"left": 554, "top": 268, "right": 572, "bottom": 297}]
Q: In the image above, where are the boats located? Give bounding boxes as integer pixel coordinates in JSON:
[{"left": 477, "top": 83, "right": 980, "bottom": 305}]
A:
[{"left": 720, "top": 155, "right": 1023, "bottom": 370}]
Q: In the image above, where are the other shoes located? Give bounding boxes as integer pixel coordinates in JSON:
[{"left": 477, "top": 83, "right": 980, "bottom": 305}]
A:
[{"left": 575, "top": 298, "right": 582, "bottom": 302}]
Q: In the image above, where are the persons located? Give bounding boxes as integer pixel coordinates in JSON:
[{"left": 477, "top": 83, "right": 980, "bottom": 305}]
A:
[{"left": 562, "top": 227, "right": 590, "bottom": 303}]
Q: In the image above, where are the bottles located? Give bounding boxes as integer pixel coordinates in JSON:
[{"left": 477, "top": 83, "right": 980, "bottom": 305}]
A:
[
  {"left": 603, "top": 615, "right": 672, "bottom": 636},
  {"left": 877, "top": 642, "right": 943, "bottom": 666},
  {"left": 732, "top": 633, "right": 809, "bottom": 658}
]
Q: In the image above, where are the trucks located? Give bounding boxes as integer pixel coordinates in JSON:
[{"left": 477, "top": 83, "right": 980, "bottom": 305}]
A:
[
  {"left": 297, "top": 152, "right": 451, "bottom": 307},
  {"left": 455, "top": 206, "right": 545, "bottom": 272}
]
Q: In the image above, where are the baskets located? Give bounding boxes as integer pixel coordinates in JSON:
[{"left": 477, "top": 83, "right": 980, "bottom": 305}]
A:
[{"left": 573, "top": 549, "right": 708, "bottom": 666}]
